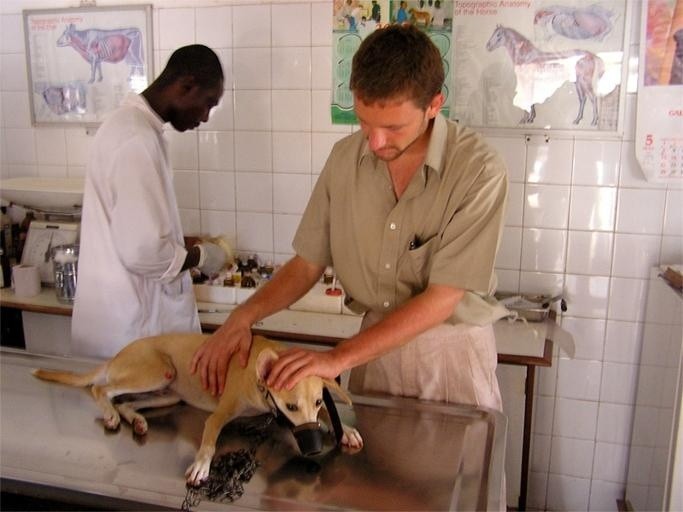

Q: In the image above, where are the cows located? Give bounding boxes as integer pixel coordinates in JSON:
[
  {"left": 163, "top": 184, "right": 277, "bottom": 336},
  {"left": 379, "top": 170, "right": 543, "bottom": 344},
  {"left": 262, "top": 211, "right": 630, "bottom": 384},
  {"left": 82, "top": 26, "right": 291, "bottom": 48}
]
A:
[{"left": 55, "top": 23, "right": 147, "bottom": 85}]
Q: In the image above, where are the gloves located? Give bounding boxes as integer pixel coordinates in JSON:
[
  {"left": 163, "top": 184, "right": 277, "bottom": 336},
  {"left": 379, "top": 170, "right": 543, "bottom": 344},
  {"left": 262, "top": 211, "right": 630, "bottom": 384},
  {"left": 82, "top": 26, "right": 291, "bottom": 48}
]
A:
[{"left": 194, "top": 237, "right": 229, "bottom": 278}]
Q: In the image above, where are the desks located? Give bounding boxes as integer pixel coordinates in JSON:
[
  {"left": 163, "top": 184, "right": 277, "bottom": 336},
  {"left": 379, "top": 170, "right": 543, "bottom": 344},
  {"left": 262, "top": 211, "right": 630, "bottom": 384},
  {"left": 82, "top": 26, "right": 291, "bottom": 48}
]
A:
[
  {"left": 0, "top": 345, "right": 508, "bottom": 512},
  {"left": 1, "top": 284, "right": 556, "bottom": 511}
]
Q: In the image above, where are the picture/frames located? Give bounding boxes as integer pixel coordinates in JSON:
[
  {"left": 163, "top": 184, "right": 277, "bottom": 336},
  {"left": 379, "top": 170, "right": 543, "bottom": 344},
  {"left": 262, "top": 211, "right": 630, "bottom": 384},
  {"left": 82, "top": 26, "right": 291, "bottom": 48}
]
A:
[
  {"left": 448, "top": 0, "right": 633, "bottom": 138},
  {"left": 23, "top": 4, "right": 154, "bottom": 127}
]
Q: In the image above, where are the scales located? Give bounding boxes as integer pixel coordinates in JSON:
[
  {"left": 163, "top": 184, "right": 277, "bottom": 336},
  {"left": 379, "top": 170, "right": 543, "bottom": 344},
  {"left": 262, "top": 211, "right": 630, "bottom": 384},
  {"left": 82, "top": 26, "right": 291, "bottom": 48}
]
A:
[{"left": 0, "top": 177, "right": 84, "bottom": 288}]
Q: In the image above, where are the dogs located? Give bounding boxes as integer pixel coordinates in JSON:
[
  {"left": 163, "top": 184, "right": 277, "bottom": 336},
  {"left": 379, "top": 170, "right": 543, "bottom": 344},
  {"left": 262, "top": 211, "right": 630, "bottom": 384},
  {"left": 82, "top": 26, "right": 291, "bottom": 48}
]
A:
[{"left": 29, "top": 331, "right": 365, "bottom": 490}]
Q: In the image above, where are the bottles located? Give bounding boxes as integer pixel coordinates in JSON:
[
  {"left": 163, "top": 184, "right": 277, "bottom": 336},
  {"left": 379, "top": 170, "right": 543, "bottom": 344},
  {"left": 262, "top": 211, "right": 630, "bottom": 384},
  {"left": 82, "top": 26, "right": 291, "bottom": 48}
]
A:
[{"left": 222, "top": 253, "right": 260, "bottom": 291}]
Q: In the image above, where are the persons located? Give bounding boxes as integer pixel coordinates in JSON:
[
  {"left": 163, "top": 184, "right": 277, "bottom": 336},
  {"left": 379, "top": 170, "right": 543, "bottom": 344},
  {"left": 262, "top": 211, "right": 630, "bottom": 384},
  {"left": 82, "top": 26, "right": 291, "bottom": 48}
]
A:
[
  {"left": 188, "top": 21, "right": 510, "bottom": 512},
  {"left": 71, "top": 43, "right": 227, "bottom": 361}
]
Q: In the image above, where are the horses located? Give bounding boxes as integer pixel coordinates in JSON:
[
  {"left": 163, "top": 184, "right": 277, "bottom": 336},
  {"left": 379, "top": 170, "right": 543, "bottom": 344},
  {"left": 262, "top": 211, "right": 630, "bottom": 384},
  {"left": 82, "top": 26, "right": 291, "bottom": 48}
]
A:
[{"left": 484, "top": 22, "right": 605, "bottom": 129}]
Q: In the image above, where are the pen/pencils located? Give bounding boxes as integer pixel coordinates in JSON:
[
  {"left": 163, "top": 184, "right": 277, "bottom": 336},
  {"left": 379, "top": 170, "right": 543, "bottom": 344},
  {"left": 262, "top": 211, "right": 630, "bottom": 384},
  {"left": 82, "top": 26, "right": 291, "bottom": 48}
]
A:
[{"left": 408, "top": 242, "right": 414, "bottom": 250}]
